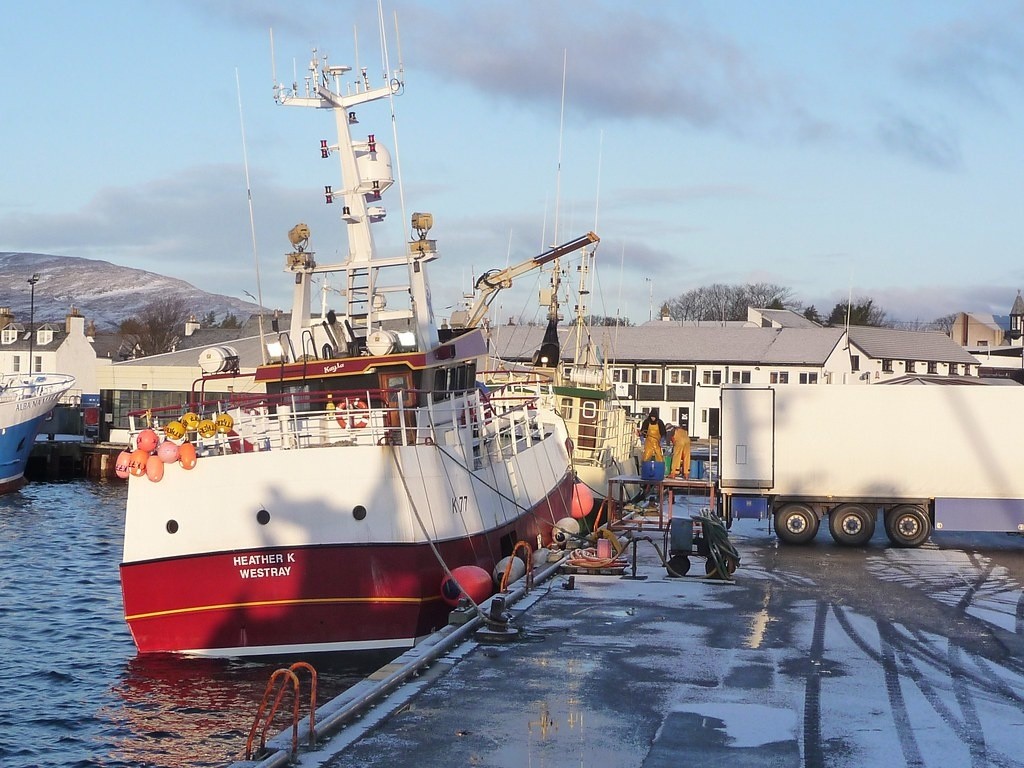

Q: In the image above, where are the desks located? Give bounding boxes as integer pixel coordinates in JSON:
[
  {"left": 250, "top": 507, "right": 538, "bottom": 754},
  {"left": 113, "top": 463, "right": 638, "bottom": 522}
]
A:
[{"left": 608, "top": 475, "right": 716, "bottom": 533}]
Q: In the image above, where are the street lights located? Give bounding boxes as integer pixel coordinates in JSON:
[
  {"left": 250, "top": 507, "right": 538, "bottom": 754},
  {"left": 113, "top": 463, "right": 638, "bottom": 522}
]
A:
[{"left": 29, "top": 273, "right": 41, "bottom": 376}]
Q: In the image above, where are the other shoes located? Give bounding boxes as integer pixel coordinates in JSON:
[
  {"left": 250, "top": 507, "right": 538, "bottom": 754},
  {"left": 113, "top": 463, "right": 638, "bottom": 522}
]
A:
[
  {"left": 684, "top": 475, "right": 689, "bottom": 480},
  {"left": 666, "top": 473, "right": 676, "bottom": 479}
]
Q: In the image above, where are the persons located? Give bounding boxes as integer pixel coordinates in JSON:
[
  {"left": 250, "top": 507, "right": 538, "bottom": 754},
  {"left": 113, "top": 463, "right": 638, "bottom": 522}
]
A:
[
  {"left": 635, "top": 408, "right": 666, "bottom": 462},
  {"left": 663, "top": 423, "right": 691, "bottom": 481}
]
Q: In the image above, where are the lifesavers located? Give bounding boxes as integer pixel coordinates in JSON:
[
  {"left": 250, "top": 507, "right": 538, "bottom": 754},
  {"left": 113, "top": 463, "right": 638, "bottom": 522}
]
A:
[{"left": 335, "top": 398, "right": 369, "bottom": 429}]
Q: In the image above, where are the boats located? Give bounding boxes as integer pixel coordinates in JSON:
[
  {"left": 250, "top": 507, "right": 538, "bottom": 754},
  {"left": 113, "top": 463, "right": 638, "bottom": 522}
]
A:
[
  {"left": 0, "top": 370, "right": 76, "bottom": 493},
  {"left": 117, "top": 5, "right": 647, "bottom": 660}
]
